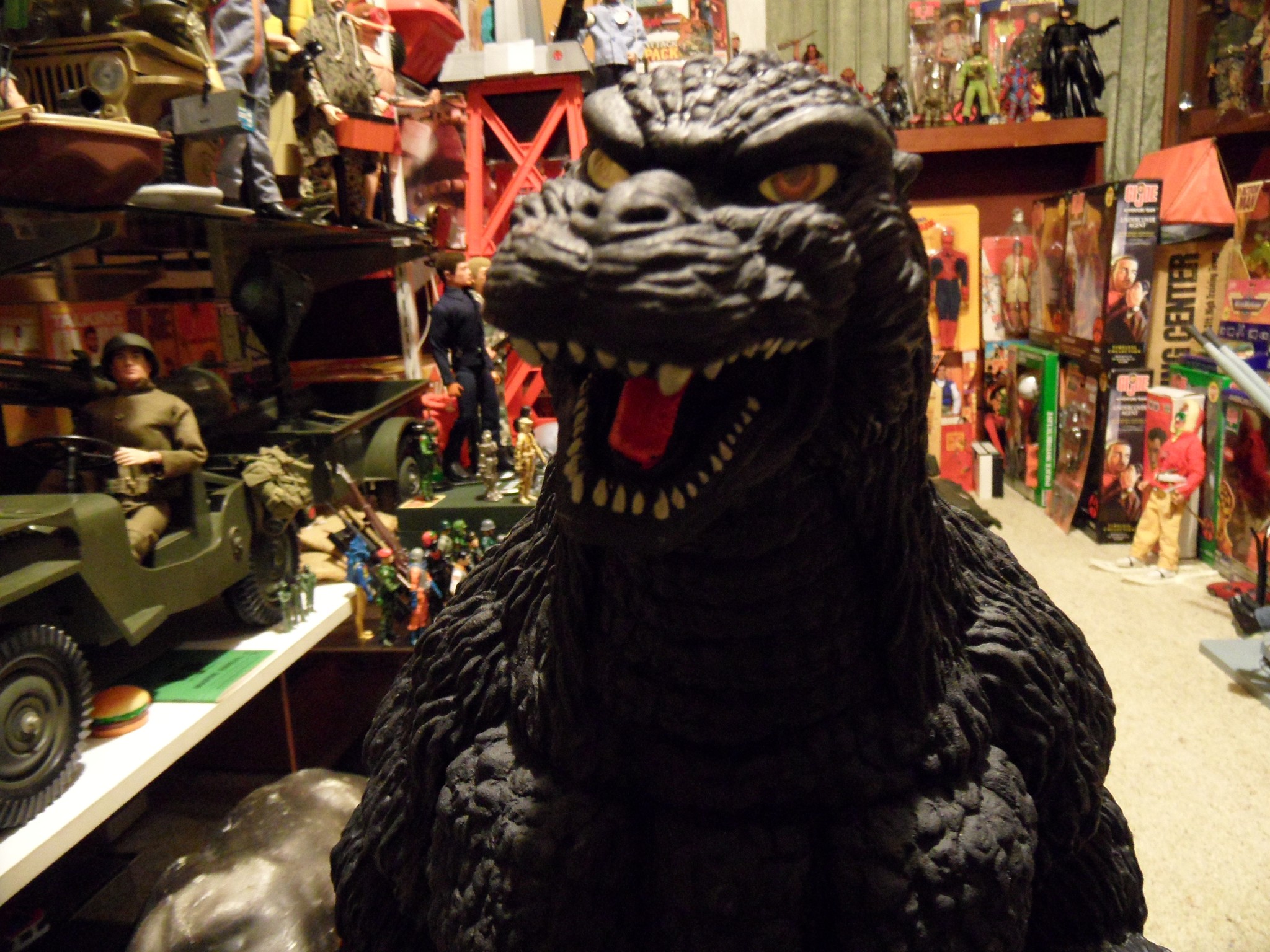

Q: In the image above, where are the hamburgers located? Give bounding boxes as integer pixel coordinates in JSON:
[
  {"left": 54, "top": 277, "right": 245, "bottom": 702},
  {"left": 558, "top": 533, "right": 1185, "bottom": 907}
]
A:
[{"left": 87, "top": 686, "right": 152, "bottom": 738}]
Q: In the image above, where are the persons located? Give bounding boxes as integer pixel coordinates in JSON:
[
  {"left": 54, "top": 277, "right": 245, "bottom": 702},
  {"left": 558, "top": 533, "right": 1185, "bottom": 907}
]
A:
[
  {"left": 34, "top": 334, "right": 208, "bottom": 566},
  {"left": 162, "top": 1, "right": 643, "bottom": 643},
  {"left": 756, "top": 1, "right": 1270, "bottom": 588}
]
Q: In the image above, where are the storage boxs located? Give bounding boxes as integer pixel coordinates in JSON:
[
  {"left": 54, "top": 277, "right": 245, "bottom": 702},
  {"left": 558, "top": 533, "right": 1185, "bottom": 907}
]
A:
[
  {"left": 471, "top": 1, "right": 732, "bottom": 74},
  {"left": 385, "top": 2, "right": 466, "bottom": 85},
  {"left": 903, "top": 1, "right": 1069, "bottom": 125},
  {"left": 903, "top": 136, "right": 1270, "bottom": 611}
]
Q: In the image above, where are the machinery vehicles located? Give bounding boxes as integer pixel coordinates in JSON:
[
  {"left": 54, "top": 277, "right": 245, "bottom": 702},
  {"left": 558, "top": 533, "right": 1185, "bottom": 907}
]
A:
[
  {"left": 177, "top": 360, "right": 431, "bottom": 517},
  {"left": 1, "top": 428, "right": 299, "bottom": 832}
]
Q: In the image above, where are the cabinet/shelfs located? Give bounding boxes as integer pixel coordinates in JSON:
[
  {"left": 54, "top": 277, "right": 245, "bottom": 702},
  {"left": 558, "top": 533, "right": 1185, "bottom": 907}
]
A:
[{"left": 0, "top": 90, "right": 451, "bottom": 952}]
